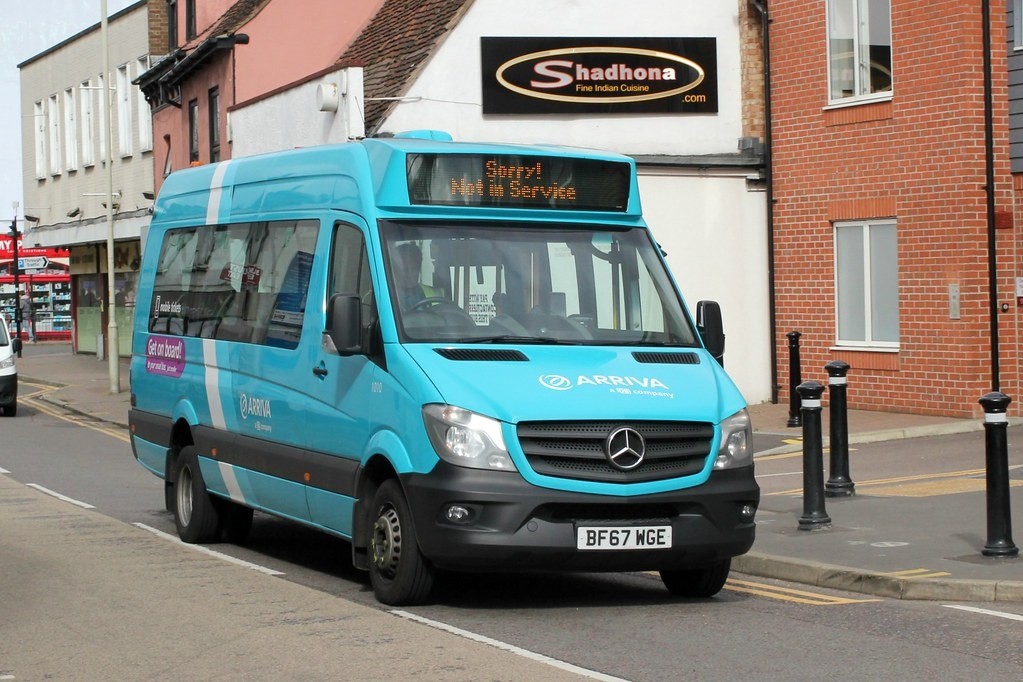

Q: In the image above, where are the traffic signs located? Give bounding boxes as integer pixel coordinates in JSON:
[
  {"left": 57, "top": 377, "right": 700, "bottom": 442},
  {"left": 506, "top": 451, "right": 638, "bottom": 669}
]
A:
[{"left": 16, "top": 256, "right": 49, "bottom": 270}]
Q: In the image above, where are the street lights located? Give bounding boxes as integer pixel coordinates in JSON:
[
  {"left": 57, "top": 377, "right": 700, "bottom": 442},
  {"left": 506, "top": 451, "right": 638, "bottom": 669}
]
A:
[{"left": 7, "top": 200, "right": 22, "bottom": 359}]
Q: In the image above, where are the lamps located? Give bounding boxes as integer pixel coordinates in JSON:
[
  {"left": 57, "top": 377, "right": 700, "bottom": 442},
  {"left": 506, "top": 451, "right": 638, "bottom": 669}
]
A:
[
  {"left": 24, "top": 215, "right": 39, "bottom": 222},
  {"left": 101, "top": 202, "right": 119, "bottom": 209},
  {"left": 66, "top": 207, "right": 80, "bottom": 217},
  {"left": 142, "top": 191, "right": 154, "bottom": 199}
]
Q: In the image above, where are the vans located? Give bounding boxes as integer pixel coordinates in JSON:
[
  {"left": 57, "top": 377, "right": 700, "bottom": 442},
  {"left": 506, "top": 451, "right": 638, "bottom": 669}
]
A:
[
  {"left": 0, "top": 312, "right": 23, "bottom": 417},
  {"left": 126, "top": 130, "right": 761, "bottom": 607}
]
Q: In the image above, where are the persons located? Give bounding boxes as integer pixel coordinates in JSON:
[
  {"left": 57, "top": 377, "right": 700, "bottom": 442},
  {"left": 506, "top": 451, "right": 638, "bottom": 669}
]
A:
[
  {"left": 115, "top": 288, "right": 129, "bottom": 307},
  {"left": 78, "top": 287, "right": 101, "bottom": 307},
  {"left": 17, "top": 290, "right": 37, "bottom": 344},
  {"left": 364, "top": 243, "right": 444, "bottom": 344}
]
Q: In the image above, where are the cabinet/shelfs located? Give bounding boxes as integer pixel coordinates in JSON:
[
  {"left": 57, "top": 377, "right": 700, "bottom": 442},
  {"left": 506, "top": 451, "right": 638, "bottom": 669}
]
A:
[{"left": 0, "top": 275, "right": 71, "bottom": 336}]
